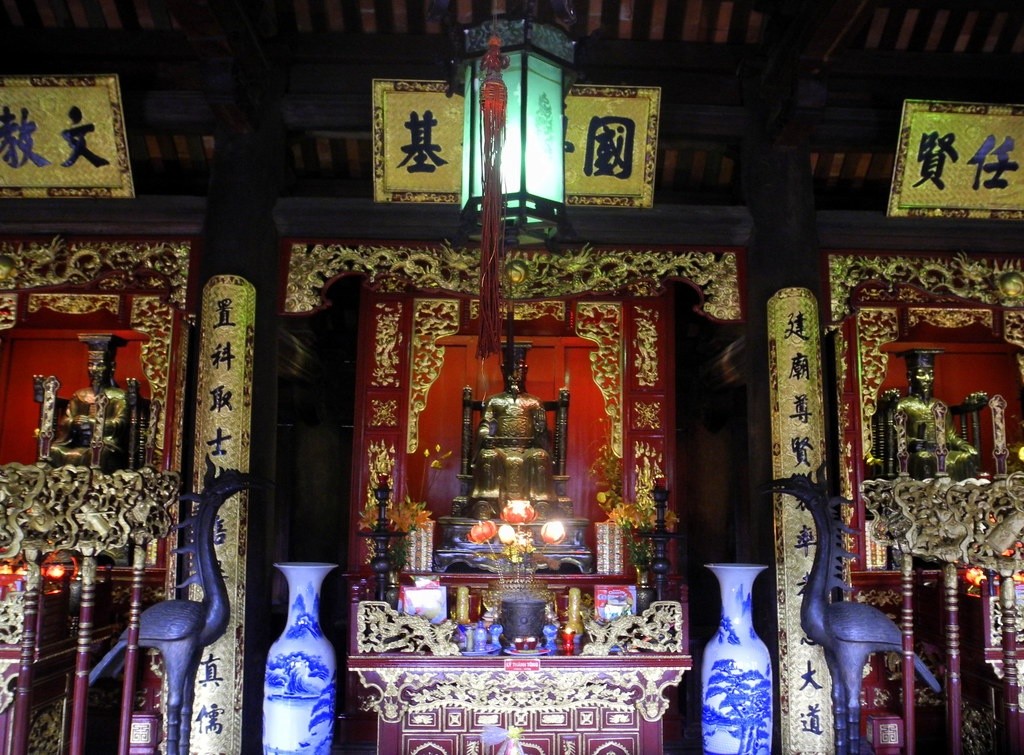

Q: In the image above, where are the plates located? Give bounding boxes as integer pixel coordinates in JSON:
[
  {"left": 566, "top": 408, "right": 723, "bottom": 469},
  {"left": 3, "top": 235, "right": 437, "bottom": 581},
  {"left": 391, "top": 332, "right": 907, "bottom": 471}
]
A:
[{"left": 504, "top": 649, "right": 551, "bottom": 657}]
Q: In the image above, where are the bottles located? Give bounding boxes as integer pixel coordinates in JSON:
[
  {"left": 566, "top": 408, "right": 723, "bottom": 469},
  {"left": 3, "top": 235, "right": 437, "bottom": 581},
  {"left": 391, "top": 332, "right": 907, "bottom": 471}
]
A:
[{"left": 465, "top": 619, "right": 487, "bottom": 653}]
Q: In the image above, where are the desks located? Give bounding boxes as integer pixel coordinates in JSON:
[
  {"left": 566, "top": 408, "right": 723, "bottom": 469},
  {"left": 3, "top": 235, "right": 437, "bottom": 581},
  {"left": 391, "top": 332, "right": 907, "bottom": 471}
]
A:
[
  {"left": 0, "top": 560, "right": 118, "bottom": 755},
  {"left": 347, "top": 582, "right": 692, "bottom": 755},
  {"left": 937, "top": 580, "right": 1024, "bottom": 755}
]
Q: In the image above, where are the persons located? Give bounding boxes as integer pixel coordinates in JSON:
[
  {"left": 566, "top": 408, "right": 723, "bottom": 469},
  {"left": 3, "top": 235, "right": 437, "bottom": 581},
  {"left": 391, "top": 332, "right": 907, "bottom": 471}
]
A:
[
  {"left": 471, "top": 355, "right": 560, "bottom": 502},
  {"left": 50, "top": 344, "right": 129, "bottom": 469},
  {"left": 883, "top": 355, "right": 984, "bottom": 479}
]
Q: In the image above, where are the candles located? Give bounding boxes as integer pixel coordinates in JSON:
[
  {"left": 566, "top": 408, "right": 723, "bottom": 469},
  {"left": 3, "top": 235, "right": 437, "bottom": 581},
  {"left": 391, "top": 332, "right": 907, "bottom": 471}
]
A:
[
  {"left": 1, "top": 563, "right": 65, "bottom": 591},
  {"left": 377, "top": 472, "right": 390, "bottom": 486},
  {"left": 965, "top": 567, "right": 984, "bottom": 586},
  {"left": 560, "top": 628, "right": 576, "bottom": 645},
  {"left": 653, "top": 474, "right": 666, "bottom": 488}
]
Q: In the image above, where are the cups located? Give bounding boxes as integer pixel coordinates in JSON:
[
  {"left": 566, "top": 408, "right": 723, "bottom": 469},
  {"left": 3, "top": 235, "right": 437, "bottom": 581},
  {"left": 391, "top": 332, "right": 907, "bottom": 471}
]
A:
[
  {"left": 526, "top": 641, "right": 537, "bottom": 650},
  {"left": 514, "top": 641, "right": 524, "bottom": 650}
]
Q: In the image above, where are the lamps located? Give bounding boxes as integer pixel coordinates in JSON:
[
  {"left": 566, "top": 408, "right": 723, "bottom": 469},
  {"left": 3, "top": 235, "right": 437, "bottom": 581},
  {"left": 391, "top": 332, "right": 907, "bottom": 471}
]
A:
[{"left": 467, "top": 498, "right": 566, "bottom": 583}]
